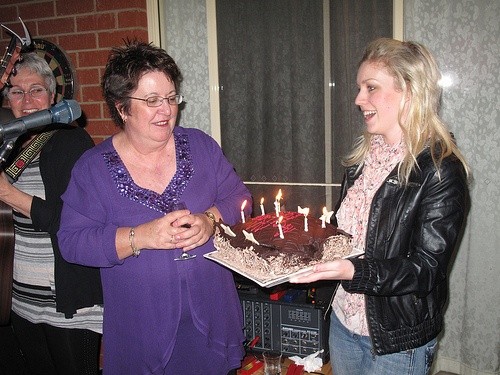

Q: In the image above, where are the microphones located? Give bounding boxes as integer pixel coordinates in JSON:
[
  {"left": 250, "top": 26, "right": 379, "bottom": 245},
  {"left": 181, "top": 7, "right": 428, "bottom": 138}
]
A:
[{"left": 1, "top": 99, "right": 82, "bottom": 138}]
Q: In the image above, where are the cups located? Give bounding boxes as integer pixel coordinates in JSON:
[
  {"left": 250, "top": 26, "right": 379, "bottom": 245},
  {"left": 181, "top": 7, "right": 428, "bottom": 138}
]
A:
[{"left": 262, "top": 349, "right": 283, "bottom": 375}]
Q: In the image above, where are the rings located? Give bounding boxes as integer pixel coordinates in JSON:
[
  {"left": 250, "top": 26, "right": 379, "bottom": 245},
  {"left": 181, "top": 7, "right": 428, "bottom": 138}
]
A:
[{"left": 170, "top": 235, "right": 175, "bottom": 241}]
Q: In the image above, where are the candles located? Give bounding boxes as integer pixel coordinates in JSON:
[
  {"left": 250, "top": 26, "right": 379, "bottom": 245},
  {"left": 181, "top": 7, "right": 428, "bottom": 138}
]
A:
[
  {"left": 321, "top": 206, "right": 327, "bottom": 228},
  {"left": 274, "top": 189, "right": 282, "bottom": 217},
  {"left": 303, "top": 207, "right": 309, "bottom": 232},
  {"left": 240, "top": 200, "right": 247, "bottom": 223},
  {"left": 277, "top": 215, "right": 284, "bottom": 239},
  {"left": 260, "top": 197, "right": 265, "bottom": 216}
]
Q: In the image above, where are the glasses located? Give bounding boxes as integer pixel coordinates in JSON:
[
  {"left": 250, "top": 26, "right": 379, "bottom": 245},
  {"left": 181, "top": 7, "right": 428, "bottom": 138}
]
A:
[
  {"left": 130, "top": 94, "right": 184, "bottom": 107},
  {"left": 8, "top": 87, "right": 49, "bottom": 99}
]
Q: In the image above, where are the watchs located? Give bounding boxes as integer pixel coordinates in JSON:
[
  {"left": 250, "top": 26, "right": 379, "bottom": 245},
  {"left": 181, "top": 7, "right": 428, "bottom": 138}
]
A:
[{"left": 205, "top": 211, "right": 216, "bottom": 235}]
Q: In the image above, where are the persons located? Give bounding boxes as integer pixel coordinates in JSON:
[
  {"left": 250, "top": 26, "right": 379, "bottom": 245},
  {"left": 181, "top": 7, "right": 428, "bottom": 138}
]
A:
[
  {"left": 56, "top": 36, "right": 254, "bottom": 375},
  {"left": 0, "top": 37, "right": 105, "bottom": 375},
  {"left": 289, "top": 38, "right": 471, "bottom": 375}
]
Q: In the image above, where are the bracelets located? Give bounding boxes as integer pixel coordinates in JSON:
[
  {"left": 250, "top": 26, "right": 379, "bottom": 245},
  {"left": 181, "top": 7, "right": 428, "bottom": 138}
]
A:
[{"left": 128, "top": 227, "right": 141, "bottom": 257}]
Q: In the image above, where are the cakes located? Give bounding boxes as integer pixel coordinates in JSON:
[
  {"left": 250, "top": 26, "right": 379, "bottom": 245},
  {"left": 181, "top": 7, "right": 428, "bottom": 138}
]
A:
[{"left": 205, "top": 210, "right": 354, "bottom": 277}]
